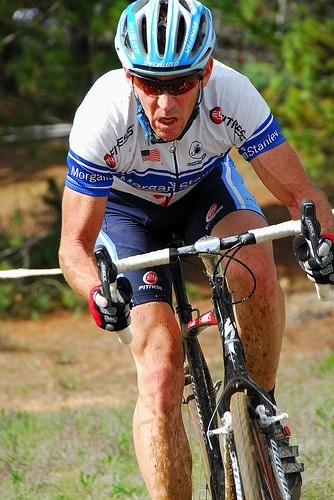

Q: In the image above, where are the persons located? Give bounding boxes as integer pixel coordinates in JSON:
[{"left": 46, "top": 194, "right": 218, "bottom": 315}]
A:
[{"left": 57, "top": 0, "right": 334, "bottom": 500}]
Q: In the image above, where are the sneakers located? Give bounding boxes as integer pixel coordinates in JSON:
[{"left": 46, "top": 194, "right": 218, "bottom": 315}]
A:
[{"left": 258, "top": 412, "right": 304, "bottom": 500}]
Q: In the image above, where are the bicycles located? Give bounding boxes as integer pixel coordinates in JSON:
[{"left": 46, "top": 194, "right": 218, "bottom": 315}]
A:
[{"left": 92, "top": 197, "right": 334, "bottom": 500}]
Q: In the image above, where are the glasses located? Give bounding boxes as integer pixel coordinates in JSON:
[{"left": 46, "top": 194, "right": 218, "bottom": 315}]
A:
[{"left": 132, "top": 73, "right": 199, "bottom": 94}]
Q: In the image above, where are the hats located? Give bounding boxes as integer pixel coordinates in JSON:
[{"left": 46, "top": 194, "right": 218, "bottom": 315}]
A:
[{"left": 114, "top": 0, "right": 216, "bottom": 79}]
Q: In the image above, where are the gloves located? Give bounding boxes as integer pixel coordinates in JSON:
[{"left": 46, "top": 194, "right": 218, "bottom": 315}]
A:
[
  {"left": 88, "top": 277, "right": 133, "bottom": 331},
  {"left": 293, "top": 234, "right": 334, "bottom": 284}
]
